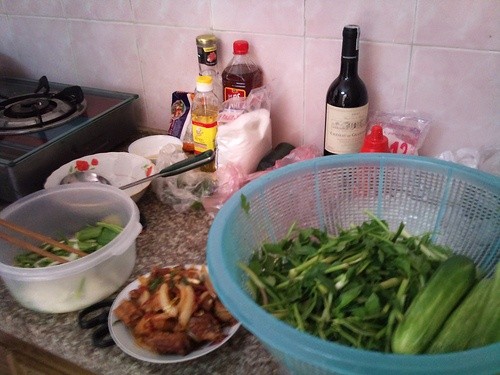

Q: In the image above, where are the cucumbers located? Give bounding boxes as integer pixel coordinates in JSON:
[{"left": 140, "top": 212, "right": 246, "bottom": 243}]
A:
[{"left": 392, "top": 254, "right": 500, "bottom": 356}]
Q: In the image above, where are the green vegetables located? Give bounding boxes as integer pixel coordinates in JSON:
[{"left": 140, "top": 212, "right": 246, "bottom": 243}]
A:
[
  {"left": 17, "top": 221, "right": 125, "bottom": 268},
  {"left": 238, "top": 194, "right": 460, "bottom": 354}
]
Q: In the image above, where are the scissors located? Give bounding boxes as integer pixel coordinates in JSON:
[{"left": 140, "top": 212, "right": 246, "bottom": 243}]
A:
[{"left": 79, "top": 298, "right": 116, "bottom": 349}]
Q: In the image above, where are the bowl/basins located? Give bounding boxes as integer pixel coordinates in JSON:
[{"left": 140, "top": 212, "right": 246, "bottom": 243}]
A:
[
  {"left": 128, "top": 135, "right": 183, "bottom": 164},
  {"left": 43, "top": 152, "right": 156, "bottom": 215},
  {"left": 0, "top": 181, "right": 142, "bottom": 314},
  {"left": 332, "top": 196, "right": 466, "bottom": 253}
]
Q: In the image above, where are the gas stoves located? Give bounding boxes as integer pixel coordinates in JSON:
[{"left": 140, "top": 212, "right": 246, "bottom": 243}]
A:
[{"left": 0, "top": 75, "right": 139, "bottom": 209}]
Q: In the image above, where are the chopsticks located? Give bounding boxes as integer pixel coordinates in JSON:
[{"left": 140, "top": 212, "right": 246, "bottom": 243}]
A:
[{"left": 0, "top": 218, "right": 90, "bottom": 264}]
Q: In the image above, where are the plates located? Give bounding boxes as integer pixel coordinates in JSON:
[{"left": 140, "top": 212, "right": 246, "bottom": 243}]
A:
[{"left": 107, "top": 263, "right": 242, "bottom": 363}]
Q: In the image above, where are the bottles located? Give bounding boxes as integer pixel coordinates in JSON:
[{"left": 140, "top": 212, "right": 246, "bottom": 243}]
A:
[
  {"left": 194, "top": 33, "right": 223, "bottom": 102},
  {"left": 221, "top": 40, "right": 262, "bottom": 120},
  {"left": 354, "top": 125, "right": 388, "bottom": 196},
  {"left": 190, "top": 76, "right": 225, "bottom": 173},
  {"left": 323, "top": 25, "right": 369, "bottom": 157}
]
